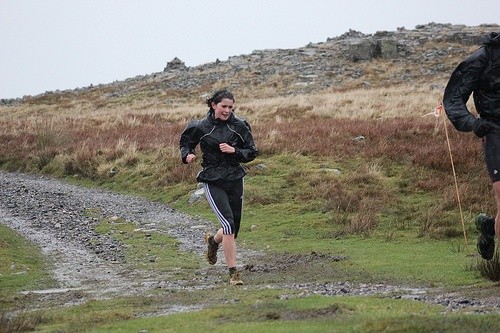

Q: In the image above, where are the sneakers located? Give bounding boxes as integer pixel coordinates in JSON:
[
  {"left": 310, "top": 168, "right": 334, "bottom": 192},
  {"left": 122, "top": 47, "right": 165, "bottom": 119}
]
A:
[
  {"left": 228, "top": 273, "right": 243, "bottom": 284},
  {"left": 474, "top": 213, "right": 494, "bottom": 261},
  {"left": 206, "top": 236, "right": 219, "bottom": 265}
]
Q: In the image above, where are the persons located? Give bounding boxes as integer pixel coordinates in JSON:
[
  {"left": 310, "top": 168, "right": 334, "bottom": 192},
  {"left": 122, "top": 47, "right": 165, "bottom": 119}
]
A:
[
  {"left": 443, "top": 32, "right": 500, "bottom": 259},
  {"left": 179, "top": 89, "right": 257, "bottom": 286}
]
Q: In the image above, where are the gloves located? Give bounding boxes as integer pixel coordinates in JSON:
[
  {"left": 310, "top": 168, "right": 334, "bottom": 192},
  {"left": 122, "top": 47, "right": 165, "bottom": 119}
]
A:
[{"left": 472, "top": 119, "right": 499, "bottom": 138}]
